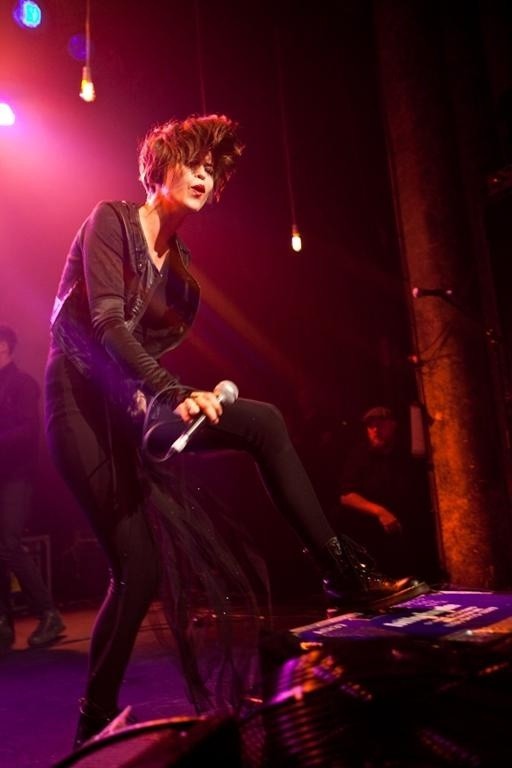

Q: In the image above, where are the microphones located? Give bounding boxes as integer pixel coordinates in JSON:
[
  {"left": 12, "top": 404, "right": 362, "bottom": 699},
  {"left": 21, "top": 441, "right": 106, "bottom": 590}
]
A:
[
  {"left": 412, "top": 286, "right": 442, "bottom": 299},
  {"left": 171, "top": 379, "right": 240, "bottom": 459}
]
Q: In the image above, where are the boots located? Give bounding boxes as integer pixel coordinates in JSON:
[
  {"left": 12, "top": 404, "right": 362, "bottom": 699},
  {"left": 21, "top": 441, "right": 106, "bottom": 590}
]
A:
[
  {"left": 74, "top": 699, "right": 122, "bottom": 751},
  {"left": 302, "top": 533, "right": 429, "bottom": 618}
]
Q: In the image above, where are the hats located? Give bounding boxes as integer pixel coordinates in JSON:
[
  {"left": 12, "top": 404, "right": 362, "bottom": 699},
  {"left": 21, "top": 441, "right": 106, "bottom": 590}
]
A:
[{"left": 363, "top": 405, "right": 390, "bottom": 423}]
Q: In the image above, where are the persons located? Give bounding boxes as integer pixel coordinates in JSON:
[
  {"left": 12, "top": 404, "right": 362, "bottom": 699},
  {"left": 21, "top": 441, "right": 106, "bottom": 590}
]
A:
[
  {"left": 0, "top": 325, "right": 70, "bottom": 647},
  {"left": 45, "top": 114, "right": 435, "bottom": 751},
  {"left": 333, "top": 405, "right": 443, "bottom": 585}
]
